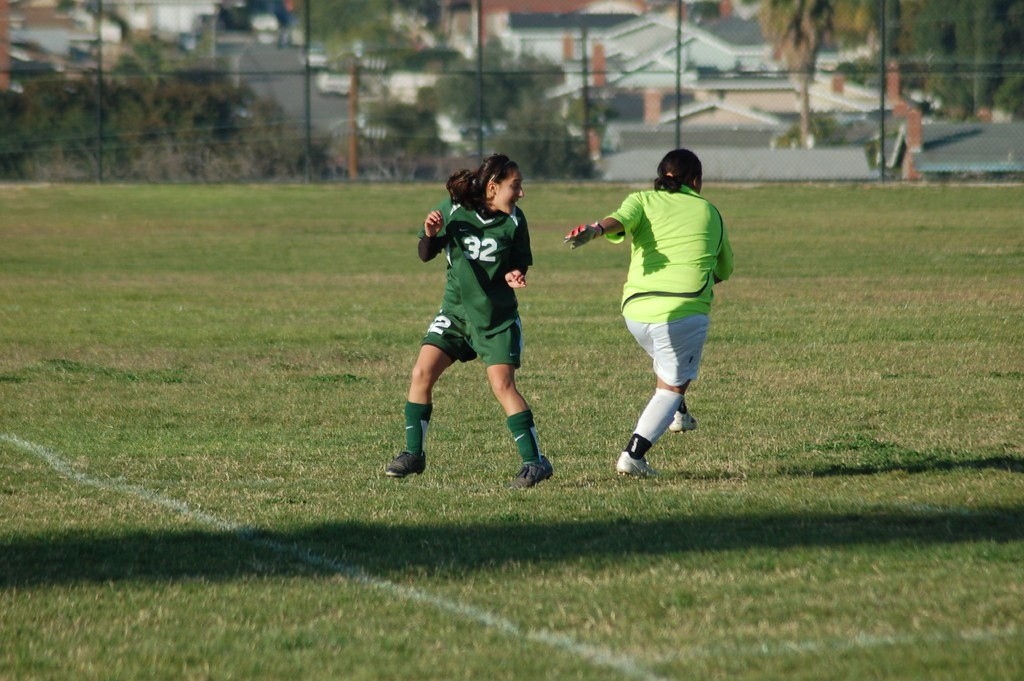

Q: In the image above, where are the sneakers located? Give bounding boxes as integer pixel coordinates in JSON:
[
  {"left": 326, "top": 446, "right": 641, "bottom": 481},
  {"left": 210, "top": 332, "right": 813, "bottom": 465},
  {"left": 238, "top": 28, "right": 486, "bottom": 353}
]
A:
[
  {"left": 508, "top": 457, "right": 554, "bottom": 491},
  {"left": 615, "top": 450, "right": 661, "bottom": 482},
  {"left": 385, "top": 449, "right": 426, "bottom": 477},
  {"left": 669, "top": 410, "right": 697, "bottom": 433}
]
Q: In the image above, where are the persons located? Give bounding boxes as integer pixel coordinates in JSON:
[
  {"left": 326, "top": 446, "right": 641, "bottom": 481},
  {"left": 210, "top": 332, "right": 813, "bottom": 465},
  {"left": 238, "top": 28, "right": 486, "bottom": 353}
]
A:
[
  {"left": 564, "top": 148, "right": 734, "bottom": 484},
  {"left": 385, "top": 152, "right": 554, "bottom": 491}
]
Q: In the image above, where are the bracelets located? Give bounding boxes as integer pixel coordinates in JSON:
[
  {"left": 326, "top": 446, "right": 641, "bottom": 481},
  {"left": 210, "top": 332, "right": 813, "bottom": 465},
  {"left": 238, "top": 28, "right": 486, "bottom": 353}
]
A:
[{"left": 591, "top": 220, "right": 605, "bottom": 239}]
